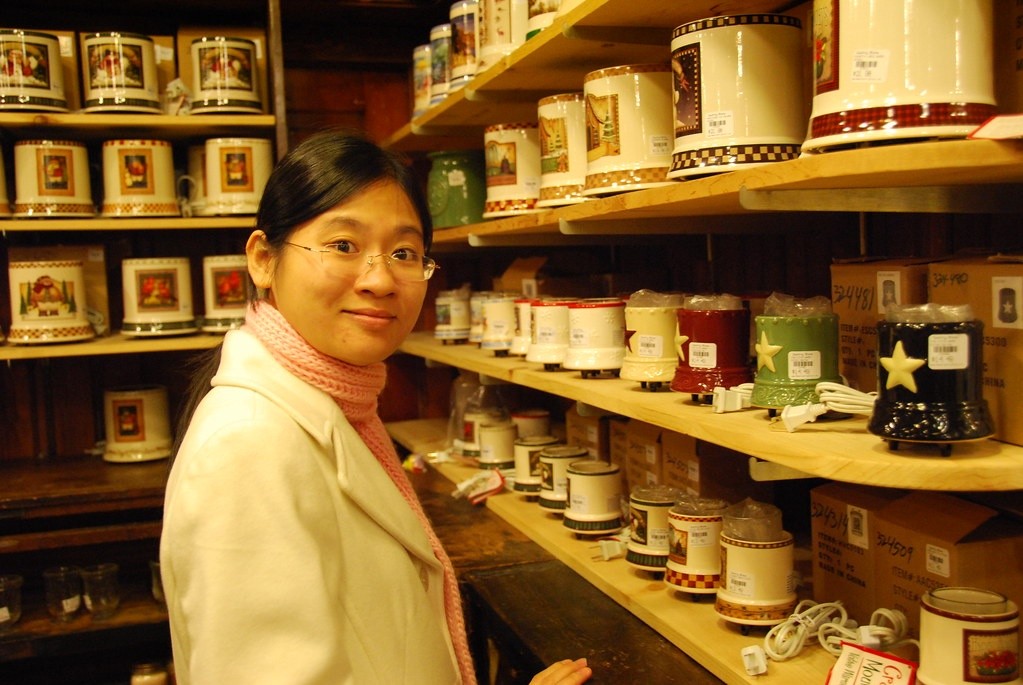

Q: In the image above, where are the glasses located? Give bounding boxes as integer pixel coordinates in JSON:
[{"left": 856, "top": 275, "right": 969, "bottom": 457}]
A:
[{"left": 280, "top": 241, "right": 440, "bottom": 283}]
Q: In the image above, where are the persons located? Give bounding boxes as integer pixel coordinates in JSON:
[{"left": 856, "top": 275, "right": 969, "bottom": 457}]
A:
[{"left": 159, "top": 131, "right": 592, "bottom": 685}]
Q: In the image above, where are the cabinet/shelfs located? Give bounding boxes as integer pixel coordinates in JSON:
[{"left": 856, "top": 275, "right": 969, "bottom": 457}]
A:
[{"left": 0, "top": 0, "right": 1023, "bottom": 685}]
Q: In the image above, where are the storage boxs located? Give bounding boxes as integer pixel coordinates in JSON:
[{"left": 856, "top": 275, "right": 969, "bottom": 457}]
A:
[
  {"left": 287, "top": 113, "right": 361, "bottom": 134},
  {"left": 812, "top": 481, "right": 910, "bottom": 626},
  {"left": 926, "top": 250, "right": 1023, "bottom": 446},
  {"left": 589, "top": 273, "right": 642, "bottom": 298},
  {"left": 565, "top": 404, "right": 624, "bottom": 463},
  {"left": 498, "top": 256, "right": 602, "bottom": 300},
  {"left": 609, "top": 418, "right": 660, "bottom": 495},
  {"left": 829, "top": 254, "right": 958, "bottom": 394},
  {"left": 284, "top": 69, "right": 363, "bottom": 111},
  {"left": 660, "top": 429, "right": 775, "bottom": 506},
  {"left": 870, "top": 489, "right": 1023, "bottom": 658},
  {"left": 739, "top": 292, "right": 815, "bottom": 357}
]
